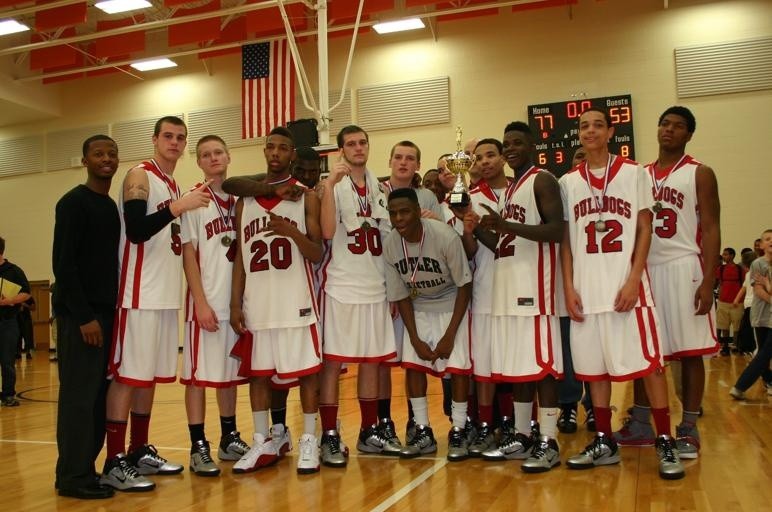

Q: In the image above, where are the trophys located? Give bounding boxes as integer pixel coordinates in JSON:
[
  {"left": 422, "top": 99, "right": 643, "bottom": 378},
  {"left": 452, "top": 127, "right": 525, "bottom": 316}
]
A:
[{"left": 444, "top": 125, "right": 474, "bottom": 210}]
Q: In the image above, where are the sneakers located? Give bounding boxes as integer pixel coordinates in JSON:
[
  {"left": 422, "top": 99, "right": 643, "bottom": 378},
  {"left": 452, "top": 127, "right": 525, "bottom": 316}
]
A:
[
  {"left": 480, "top": 432, "right": 537, "bottom": 461},
  {"left": 378, "top": 417, "right": 401, "bottom": 446},
  {"left": 0, "top": 397, "right": 20, "bottom": 406},
  {"left": 189, "top": 439, "right": 221, "bottom": 478},
  {"left": 405, "top": 419, "right": 438, "bottom": 446},
  {"left": 731, "top": 343, "right": 737, "bottom": 351},
  {"left": 520, "top": 435, "right": 561, "bottom": 474},
  {"left": 127, "top": 443, "right": 185, "bottom": 476},
  {"left": 320, "top": 429, "right": 347, "bottom": 468},
  {"left": 531, "top": 419, "right": 540, "bottom": 435},
  {"left": 465, "top": 419, "right": 477, "bottom": 443},
  {"left": 98, "top": 451, "right": 156, "bottom": 493},
  {"left": 48, "top": 353, "right": 57, "bottom": 361},
  {"left": 556, "top": 407, "right": 578, "bottom": 433},
  {"left": 231, "top": 432, "right": 278, "bottom": 474},
  {"left": 356, "top": 423, "right": 403, "bottom": 455},
  {"left": 217, "top": 430, "right": 251, "bottom": 462},
  {"left": 729, "top": 386, "right": 745, "bottom": 400},
  {"left": 611, "top": 407, "right": 656, "bottom": 446},
  {"left": 468, "top": 421, "right": 494, "bottom": 458},
  {"left": 446, "top": 426, "right": 469, "bottom": 461},
  {"left": 318, "top": 437, "right": 350, "bottom": 459},
  {"left": 675, "top": 418, "right": 701, "bottom": 460},
  {"left": 565, "top": 431, "right": 620, "bottom": 470},
  {"left": 268, "top": 423, "right": 293, "bottom": 462},
  {"left": 720, "top": 346, "right": 730, "bottom": 355},
  {"left": 584, "top": 409, "right": 595, "bottom": 432},
  {"left": 495, "top": 415, "right": 514, "bottom": 442},
  {"left": 18, "top": 355, "right": 22, "bottom": 360},
  {"left": 398, "top": 425, "right": 438, "bottom": 459},
  {"left": 656, "top": 434, "right": 686, "bottom": 480},
  {"left": 764, "top": 381, "right": 772, "bottom": 394},
  {"left": 27, "top": 353, "right": 32, "bottom": 359},
  {"left": 744, "top": 352, "right": 754, "bottom": 363},
  {"left": 296, "top": 433, "right": 321, "bottom": 474}
]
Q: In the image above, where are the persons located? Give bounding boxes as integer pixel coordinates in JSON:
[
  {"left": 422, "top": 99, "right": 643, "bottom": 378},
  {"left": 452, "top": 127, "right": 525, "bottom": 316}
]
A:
[
  {"left": 612, "top": 106, "right": 721, "bottom": 460},
  {"left": 464, "top": 138, "right": 486, "bottom": 446},
  {"left": 383, "top": 187, "right": 472, "bottom": 462},
  {"left": 220, "top": 146, "right": 348, "bottom": 465},
  {"left": 478, "top": 121, "right": 565, "bottom": 472},
  {"left": 714, "top": 254, "right": 723, "bottom": 310},
  {"left": 422, "top": 169, "right": 462, "bottom": 422},
  {"left": 557, "top": 108, "right": 686, "bottom": 481},
  {"left": 14, "top": 295, "right": 37, "bottom": 359},
  {"left": 734, "top": 252, "right": 757, "bottom": 356},
  {"left": 729, "top": 229, "right": 772, "bottom": 400},
  {"left": 179, "top": 136, "right": 253, "bottom": 478},
  {"left": 49, "top": 135, "right": 122, "bottom": 500},
  {"left": 379, "top": 141, "right": 446, "bottom": 448},
  {"left": 0, "top": 237, "right": 32, "bottom": 406},
  {"left": 320, "top": 125, "right": 402, "bottom": 470},
  {"left": 466, "top": 139, "right": 512, "bottom": 461},
  {"left": 715, "top": 248, "right": 746, "bottom": 357},
  {"left": 99, "top": 116, "right": 214, "bottom": 492},
  {"left": 227, "top": 128, "right": 322, "bottom": 475},
  {"left": 754, "top": 238, "right": 765, "bottom": 257},
  {"left": 736, "top": 248, "right": 753, "bottom": 285},
  {"left": 571, "top": 146, "right": 590, "bottom": 169},
  {"left": 438, "top": 154, "right": 488, "bottom": 443}
]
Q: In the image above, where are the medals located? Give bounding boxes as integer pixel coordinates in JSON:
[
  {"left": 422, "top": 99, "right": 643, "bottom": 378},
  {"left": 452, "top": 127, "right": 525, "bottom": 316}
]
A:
[
  {"left": 203, "top": 180, "right": 232, "bottom": 246},
  {"left": 348, "top": 173, "right": 371, "bottom": 232},
  {"left": 652, "top": 154, "right": 688, "bottom": 213},
  {"left": 151, "top": 159, "right": 181, "bottom": 234},
  {"left": 489, "top": 180, "right": 512, "bottom": 204},
  {"left": 501, "top": 165, "right": 535, "bottom": 220},
  {"left": 585, "top": 152, "right": 612, "bottom": 232},
  {"left": 402, "top": 225, "right": 425, "bottom": 299}
]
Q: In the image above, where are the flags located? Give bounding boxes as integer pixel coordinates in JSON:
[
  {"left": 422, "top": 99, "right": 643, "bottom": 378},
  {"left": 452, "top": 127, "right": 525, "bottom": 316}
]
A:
[{"left": 241, "top": 42, "right": 296, "bottom": 140}]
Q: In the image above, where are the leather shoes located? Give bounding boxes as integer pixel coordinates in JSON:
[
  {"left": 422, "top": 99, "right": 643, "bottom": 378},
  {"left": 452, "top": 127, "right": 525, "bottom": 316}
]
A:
[
  {"left": 54, "top": 473, "right": 101, "bottom": 489},
  {"left": 58, "top": 485, "right": 115, "bottom": 500}
]
